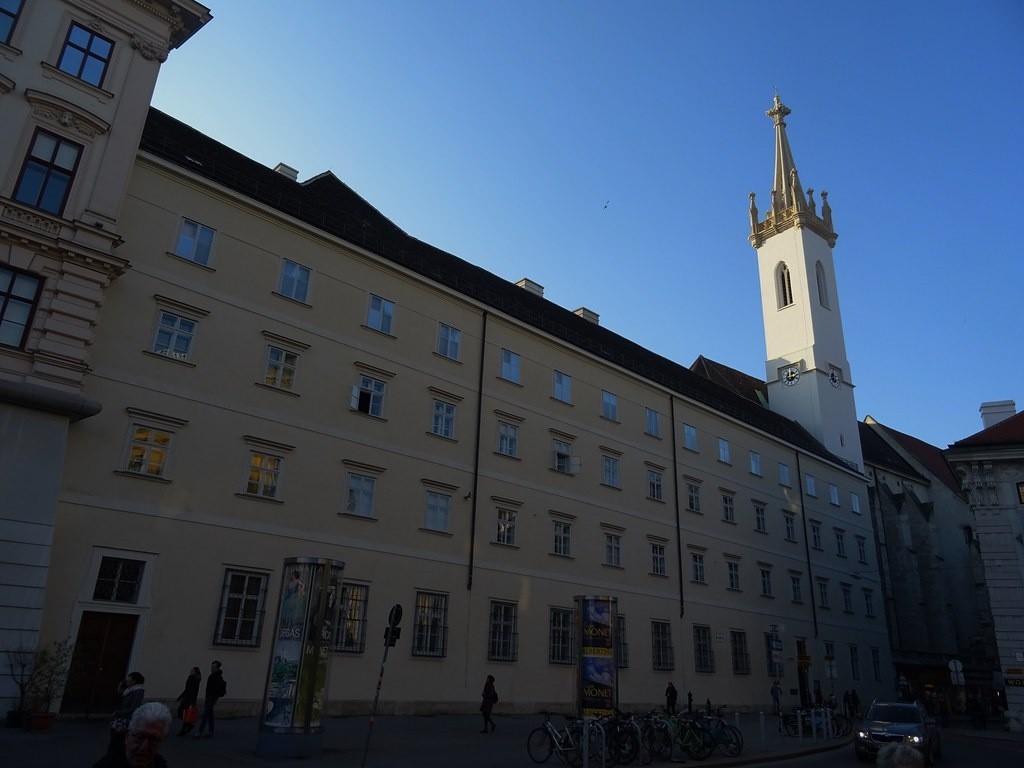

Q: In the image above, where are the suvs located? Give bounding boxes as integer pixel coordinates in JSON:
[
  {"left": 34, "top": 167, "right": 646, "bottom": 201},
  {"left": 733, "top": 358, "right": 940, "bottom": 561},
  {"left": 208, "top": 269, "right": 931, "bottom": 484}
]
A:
[{"left": 854, "top": 695, "right": 940, "bottom": 766}]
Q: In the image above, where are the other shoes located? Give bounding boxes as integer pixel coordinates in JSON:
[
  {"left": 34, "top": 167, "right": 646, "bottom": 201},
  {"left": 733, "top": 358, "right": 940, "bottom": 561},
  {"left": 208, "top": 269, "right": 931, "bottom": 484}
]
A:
[
  {"left": 192, "top": 732, "right": 202, "bottom": 739},
  {"left": 176, "top": 728, "right": 185, "bottom": 737},
  {"left": 491, "top": 723, "right": 496, "bottom": 732},
  {"left": 480, "top": 728, "right": 488, "bottom": 733},
  {"left": 203, "top": 731, "right": 215, "bottom": 738},
  {"left": 773, "top": 712, "right": 779, "bottom": 716},
  {"left": 186, "top": 724, "right": 194, "bottom": 735}
]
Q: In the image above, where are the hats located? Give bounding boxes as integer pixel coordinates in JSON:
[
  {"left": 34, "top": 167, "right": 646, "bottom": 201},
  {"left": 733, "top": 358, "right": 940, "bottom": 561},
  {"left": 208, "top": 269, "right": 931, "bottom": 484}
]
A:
[
  {"left": 487, "top": 675, "right": 495, "bottom": 681},
  {"left": 129, "top": 671, "right": 145, "bottom": 684}
]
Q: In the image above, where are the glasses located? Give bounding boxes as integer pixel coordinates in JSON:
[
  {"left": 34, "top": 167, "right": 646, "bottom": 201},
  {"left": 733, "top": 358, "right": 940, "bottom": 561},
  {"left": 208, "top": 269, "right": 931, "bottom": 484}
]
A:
[{"left": 131, "top": 733, "right": 165, "bottom": 745}]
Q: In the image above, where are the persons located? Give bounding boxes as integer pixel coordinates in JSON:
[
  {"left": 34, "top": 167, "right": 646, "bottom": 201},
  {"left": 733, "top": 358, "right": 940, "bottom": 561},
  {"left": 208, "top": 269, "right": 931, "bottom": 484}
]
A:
[
  {"left": 771, "top": 681, "right": 783, "bottom": 716},
  {"left": 90, "top": 672, "right": 172, "bottom": 768},
  {"left": 193, "top": 660, "right": 224, "bottom": 739},
  {"left": 665, "top": 682, "right": 678, "bottom": 718},
  {"left": 479, "top": 675, "right": 498, "bottom": 733},
  {"left": 843, "top": 688, "right": 861, "bottom": 715},
  {"left": 175, "top": 667, "right": 201, "bottom": 737},
  {"left": 875, "top": 741, "right": 924, "bottom": 768}
]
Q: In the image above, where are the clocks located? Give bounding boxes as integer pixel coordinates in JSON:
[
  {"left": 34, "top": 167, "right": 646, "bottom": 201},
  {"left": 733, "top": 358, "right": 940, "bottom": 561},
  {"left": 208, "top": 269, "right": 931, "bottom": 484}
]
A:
[
  {"left": 829, "top": 369, "right": 840, "bottom": 388},
  {"left": 781, "top": 366, "right": 800, "bottom": 386}
]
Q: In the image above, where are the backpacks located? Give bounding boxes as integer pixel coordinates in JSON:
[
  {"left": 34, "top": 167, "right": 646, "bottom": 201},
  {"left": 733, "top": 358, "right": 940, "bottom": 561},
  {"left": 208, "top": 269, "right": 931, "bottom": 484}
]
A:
[{"left": 219, "top": 679, "right": 227, "bottom": 697}]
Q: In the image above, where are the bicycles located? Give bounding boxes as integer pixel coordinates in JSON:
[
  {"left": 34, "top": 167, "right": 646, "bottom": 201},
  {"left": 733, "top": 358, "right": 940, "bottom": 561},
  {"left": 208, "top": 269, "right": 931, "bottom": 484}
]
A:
[
  {"left": 525, "top": 703, "right": 744, "bottom": 767},
  {"left": 779, "top": 706, "right": 853, "bottom": 739}
]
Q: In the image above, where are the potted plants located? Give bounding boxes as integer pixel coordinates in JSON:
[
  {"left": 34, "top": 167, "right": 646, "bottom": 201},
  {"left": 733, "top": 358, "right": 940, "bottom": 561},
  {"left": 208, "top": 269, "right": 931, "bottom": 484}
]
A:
[{"left": 4, "top": 637, "right": 76, "bottom": 731}]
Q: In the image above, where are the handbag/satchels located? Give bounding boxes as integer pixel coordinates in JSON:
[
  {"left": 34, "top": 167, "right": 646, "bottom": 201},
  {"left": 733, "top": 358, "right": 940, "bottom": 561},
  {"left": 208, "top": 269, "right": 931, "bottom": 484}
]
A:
[
  {"left": 105, "top": 716, "right": 130, "bottom": 735},
  {"left": 183, "top": 704, "right": 199, "bottom": 723},
  {"left": 492, "top": 692, "right": 498, "bottom": 704}
]
